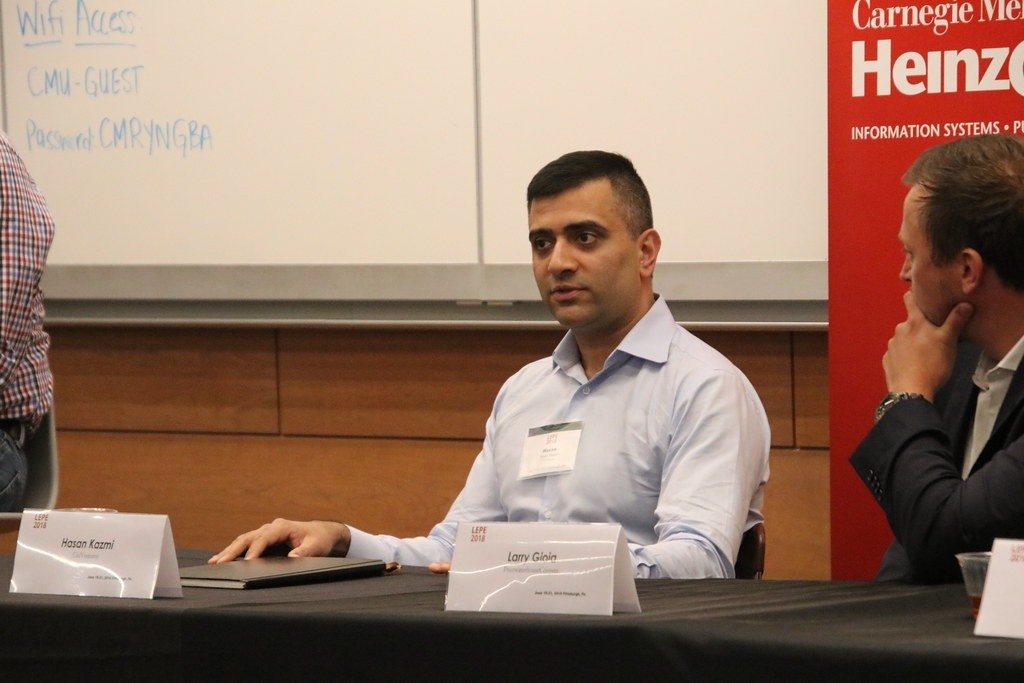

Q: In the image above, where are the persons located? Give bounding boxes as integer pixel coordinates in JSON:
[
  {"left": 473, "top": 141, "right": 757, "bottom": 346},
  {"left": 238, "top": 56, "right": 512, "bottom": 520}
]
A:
[
  {"left": 208, "top": 151, "right": 771, "bottom": 579},
  {"left": 0, "top": 133, "right": 56, "bottom": 514},
  {"left": 846, "top": 135, "right": 1024, "bottom": 581}
]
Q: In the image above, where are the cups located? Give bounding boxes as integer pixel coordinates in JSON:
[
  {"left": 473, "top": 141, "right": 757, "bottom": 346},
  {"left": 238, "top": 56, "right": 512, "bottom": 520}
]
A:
[{"left": 955, "top": 551, "right": 993, "bottom": 620}]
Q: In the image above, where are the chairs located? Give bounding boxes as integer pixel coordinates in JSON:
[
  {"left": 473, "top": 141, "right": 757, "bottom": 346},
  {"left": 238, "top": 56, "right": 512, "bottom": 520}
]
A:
[{"left": 0, "top": 401, "right": 59, "bottom": 524}]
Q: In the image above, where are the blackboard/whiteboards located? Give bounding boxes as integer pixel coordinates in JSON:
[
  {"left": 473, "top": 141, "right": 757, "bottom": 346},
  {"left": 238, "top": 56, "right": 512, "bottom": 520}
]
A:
[{"left": 0, "top": 0, "right": 829, "bottom": 330}]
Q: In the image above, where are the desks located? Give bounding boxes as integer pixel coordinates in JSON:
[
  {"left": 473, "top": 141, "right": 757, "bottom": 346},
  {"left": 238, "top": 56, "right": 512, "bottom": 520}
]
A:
[{"left": 0, "top": 544, "right": 1024, "bottom": 683}]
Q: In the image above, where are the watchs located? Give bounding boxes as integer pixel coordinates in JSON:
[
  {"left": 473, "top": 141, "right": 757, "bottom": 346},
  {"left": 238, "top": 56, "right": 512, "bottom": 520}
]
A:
[{"left": 876, "top": 391, "right": 924, "bottom": 423}]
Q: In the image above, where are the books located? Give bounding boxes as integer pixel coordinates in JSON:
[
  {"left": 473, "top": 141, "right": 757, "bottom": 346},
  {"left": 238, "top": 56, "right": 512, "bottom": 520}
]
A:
[{"left": 178, "top": 558, "right": 387, "bottom": 589}]
